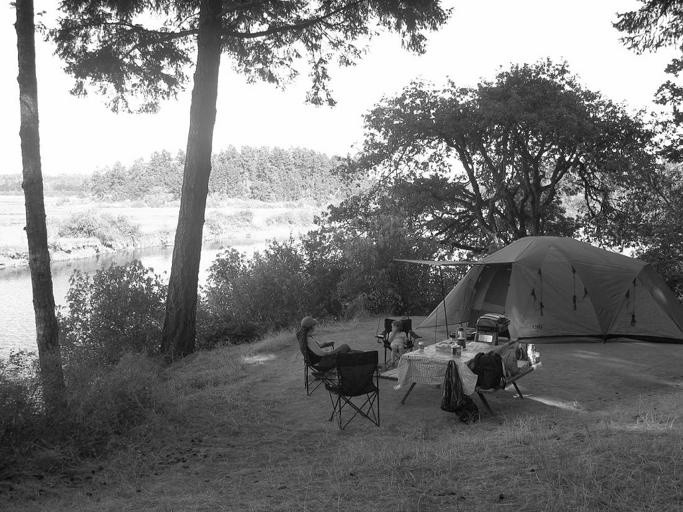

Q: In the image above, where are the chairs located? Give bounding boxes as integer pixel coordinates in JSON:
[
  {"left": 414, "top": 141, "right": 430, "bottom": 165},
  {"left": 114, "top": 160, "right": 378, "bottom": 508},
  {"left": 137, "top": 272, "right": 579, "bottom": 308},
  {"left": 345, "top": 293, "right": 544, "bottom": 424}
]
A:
[{"left": 294, "top": 318, "right": 535, "bottom": 430}]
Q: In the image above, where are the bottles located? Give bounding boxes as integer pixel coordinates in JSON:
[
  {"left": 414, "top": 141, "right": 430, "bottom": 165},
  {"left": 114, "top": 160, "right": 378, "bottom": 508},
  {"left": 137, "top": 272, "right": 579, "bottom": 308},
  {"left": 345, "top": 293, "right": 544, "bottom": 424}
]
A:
[
  {"left": 451, "top": 328, "right": 467, "bottom": 357},
  {"left": 413, "top": 338, "right": 425, "bottom": 355}
]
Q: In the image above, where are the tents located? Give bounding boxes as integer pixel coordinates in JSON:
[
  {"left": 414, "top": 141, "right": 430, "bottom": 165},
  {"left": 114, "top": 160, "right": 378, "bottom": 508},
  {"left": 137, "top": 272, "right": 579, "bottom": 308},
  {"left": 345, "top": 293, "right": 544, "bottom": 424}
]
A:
[{"left": 389, "top": 235, "right": 681, "bottom": 348}]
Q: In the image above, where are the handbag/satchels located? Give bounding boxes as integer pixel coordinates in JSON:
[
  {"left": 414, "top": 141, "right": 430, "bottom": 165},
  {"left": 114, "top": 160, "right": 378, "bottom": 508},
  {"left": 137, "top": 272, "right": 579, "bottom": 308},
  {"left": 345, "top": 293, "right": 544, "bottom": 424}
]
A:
[{"left": 468, "top": 351, "right": 505, "bottom": 390}]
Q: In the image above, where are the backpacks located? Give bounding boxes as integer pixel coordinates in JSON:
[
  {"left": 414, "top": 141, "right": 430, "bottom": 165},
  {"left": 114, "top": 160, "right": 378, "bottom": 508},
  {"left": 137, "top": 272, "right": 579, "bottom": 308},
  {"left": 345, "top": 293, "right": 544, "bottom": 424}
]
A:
[{"left": 505, "top": 343, "right": 540, "bottom": 377}]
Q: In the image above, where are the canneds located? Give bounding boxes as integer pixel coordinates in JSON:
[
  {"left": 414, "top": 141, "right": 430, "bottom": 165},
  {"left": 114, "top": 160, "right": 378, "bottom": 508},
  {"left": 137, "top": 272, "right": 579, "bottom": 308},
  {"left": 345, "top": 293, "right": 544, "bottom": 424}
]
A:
[{"left": 450, "top": 345, "right": 461, "bottom": 358}]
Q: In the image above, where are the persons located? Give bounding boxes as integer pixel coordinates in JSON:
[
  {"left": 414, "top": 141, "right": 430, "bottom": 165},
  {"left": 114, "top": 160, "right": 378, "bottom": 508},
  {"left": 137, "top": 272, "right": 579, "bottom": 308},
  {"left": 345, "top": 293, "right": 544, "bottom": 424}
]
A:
[
  {"left": 301, "top": 315, "right": 365, "bottom": 369},
  {"left": 386, "top": 320, "right": 408, "bottom": 364}
]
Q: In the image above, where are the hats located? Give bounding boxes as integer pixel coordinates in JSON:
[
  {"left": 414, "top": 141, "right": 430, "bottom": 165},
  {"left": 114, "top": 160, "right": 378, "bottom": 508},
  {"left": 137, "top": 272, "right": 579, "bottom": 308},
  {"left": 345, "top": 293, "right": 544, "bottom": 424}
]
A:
[{"left": 301, "top": 316, "right": 318, "bottom": 329}]
